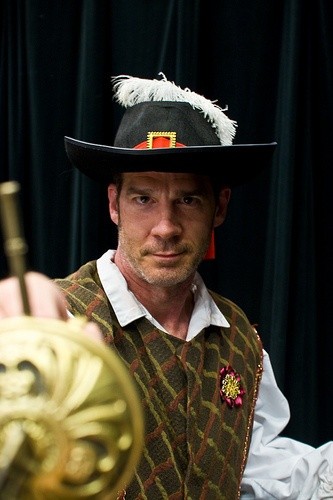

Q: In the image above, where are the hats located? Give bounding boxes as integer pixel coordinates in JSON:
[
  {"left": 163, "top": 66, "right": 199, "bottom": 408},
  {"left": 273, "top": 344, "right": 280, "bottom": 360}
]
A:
[{"left": 64, "top": 73, "right": 278, "bottom": 185}]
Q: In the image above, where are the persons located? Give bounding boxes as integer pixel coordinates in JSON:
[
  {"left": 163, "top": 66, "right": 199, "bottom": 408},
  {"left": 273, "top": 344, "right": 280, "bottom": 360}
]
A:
[{"left": 1, "top": 71, "right": 333, "bottom": 500}]
